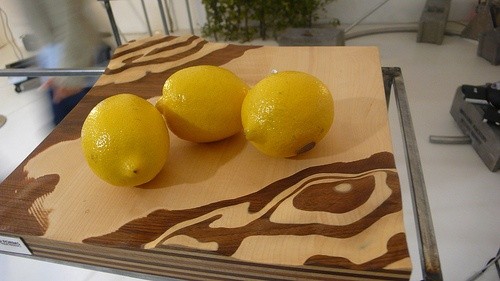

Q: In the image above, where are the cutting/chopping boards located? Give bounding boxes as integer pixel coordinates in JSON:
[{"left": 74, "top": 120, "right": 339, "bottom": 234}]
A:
[{"left": 0, "top": 35, "right": 413, "bottom": 281}]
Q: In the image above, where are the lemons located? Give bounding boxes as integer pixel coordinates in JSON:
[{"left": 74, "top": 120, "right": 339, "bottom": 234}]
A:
[
  {"left": 81, "top": 93, "right": 170, "bottom": 186},
  {"left": 241, "top": 70, "right": 334, "bottom": 158},
  {"left": 155, "top": 65, "right": 252, "bottom": 142}
]
